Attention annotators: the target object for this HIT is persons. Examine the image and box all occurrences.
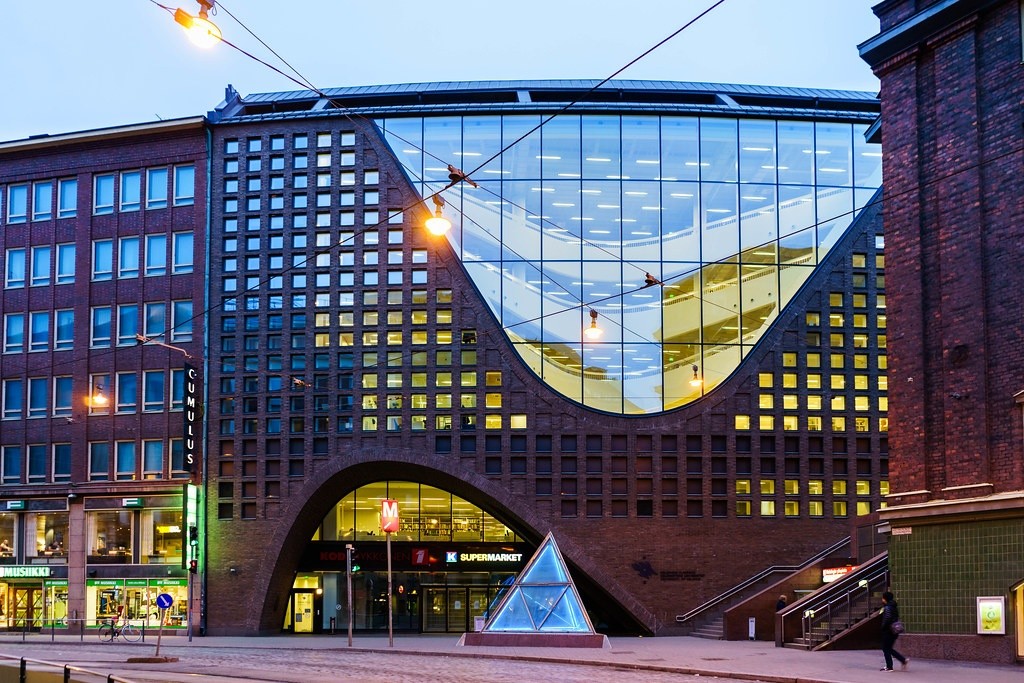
[111,610,121,643]
[0,539,11,553]
[776,594,789,612]
[49,540,61,551]
[876,590,910,672]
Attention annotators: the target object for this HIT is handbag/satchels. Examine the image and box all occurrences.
[889,615,904,634]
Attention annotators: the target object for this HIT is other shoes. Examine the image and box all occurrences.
[880,667,893,671]
[902,658,910,671]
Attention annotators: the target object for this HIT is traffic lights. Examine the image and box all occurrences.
[188,559,198,574]
[189,526,199,547]
[350,549,361,572]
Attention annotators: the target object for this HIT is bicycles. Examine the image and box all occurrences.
[98,616,142,642]
